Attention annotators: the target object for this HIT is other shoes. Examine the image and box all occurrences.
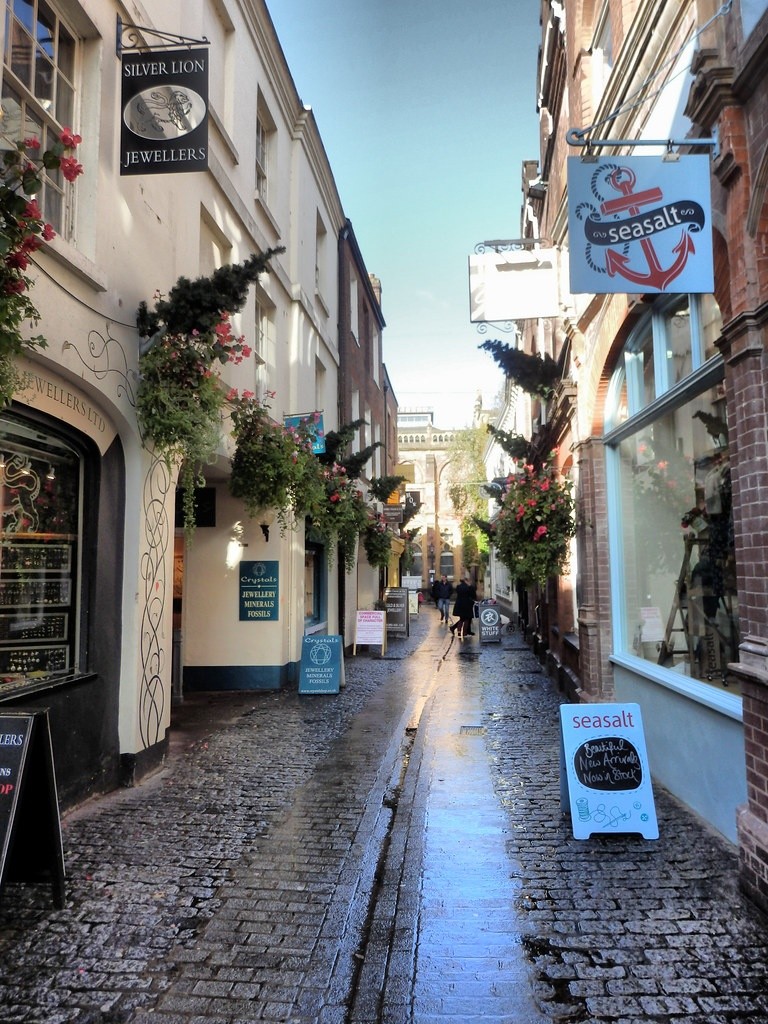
[450,627,454,634]
[458,631,461,636]
[468,632,475,635]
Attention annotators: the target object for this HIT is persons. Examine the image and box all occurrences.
[703,448,738,596]
[416,590,423,607]
[431,574,478,637]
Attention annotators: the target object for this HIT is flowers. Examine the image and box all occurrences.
[135,311,414,575]
[483,446,595,594]
[630,410,730,579]
[0,124,84,411]
